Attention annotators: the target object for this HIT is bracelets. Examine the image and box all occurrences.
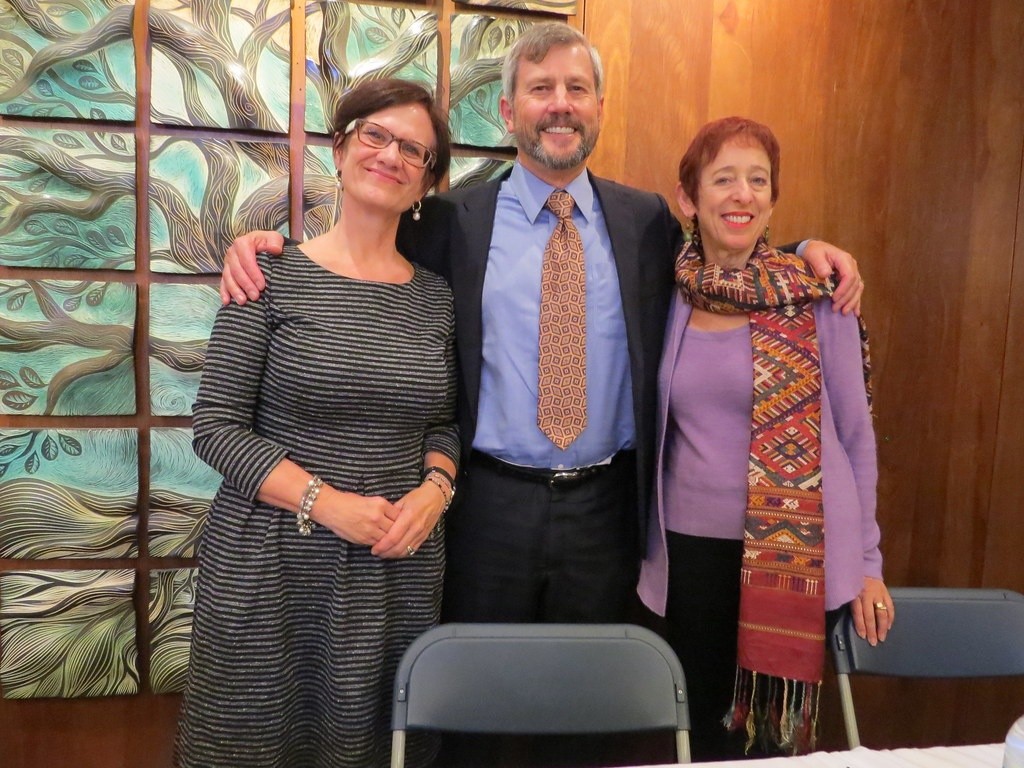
[424,475,454,516]
[423,466,456,491]
[296,474,323,536]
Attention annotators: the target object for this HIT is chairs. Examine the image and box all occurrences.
[390,623,691,768]
[827,587,1024,751]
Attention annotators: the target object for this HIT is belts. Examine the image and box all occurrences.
[469,448,625,494]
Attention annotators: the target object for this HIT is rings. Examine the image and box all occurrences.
[407,546,415,555]
[874,602,886,609]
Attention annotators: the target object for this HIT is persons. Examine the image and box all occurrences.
[221,21,864,768]
[174,78,460,767]
[636,117,894,761]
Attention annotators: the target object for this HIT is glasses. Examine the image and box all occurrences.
[334,119,438,172]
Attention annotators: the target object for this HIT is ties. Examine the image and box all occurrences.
[537,190,587,451]
